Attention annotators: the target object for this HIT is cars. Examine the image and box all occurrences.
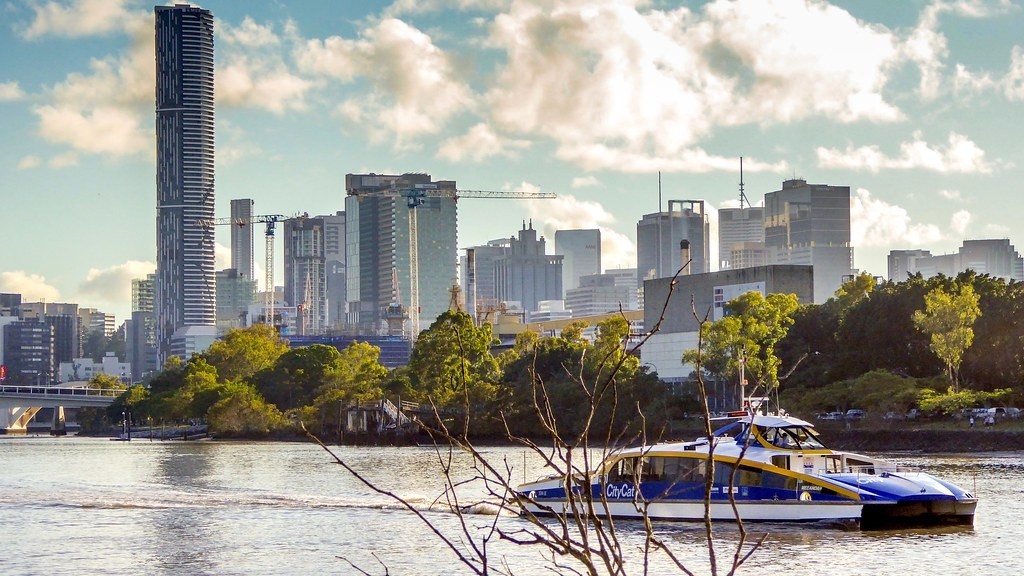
[817,406,1024,421]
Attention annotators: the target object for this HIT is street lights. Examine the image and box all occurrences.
[150,370,153,378]
[129,372,132,387]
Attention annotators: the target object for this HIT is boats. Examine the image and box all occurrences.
[515,343,979,532]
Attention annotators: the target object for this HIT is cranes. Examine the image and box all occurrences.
[346,186,557,358]
[192,211,309,336]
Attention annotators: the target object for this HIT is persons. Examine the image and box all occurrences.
[983,415,994,430]
[969,416,974,430]
[777,432,790,449]
